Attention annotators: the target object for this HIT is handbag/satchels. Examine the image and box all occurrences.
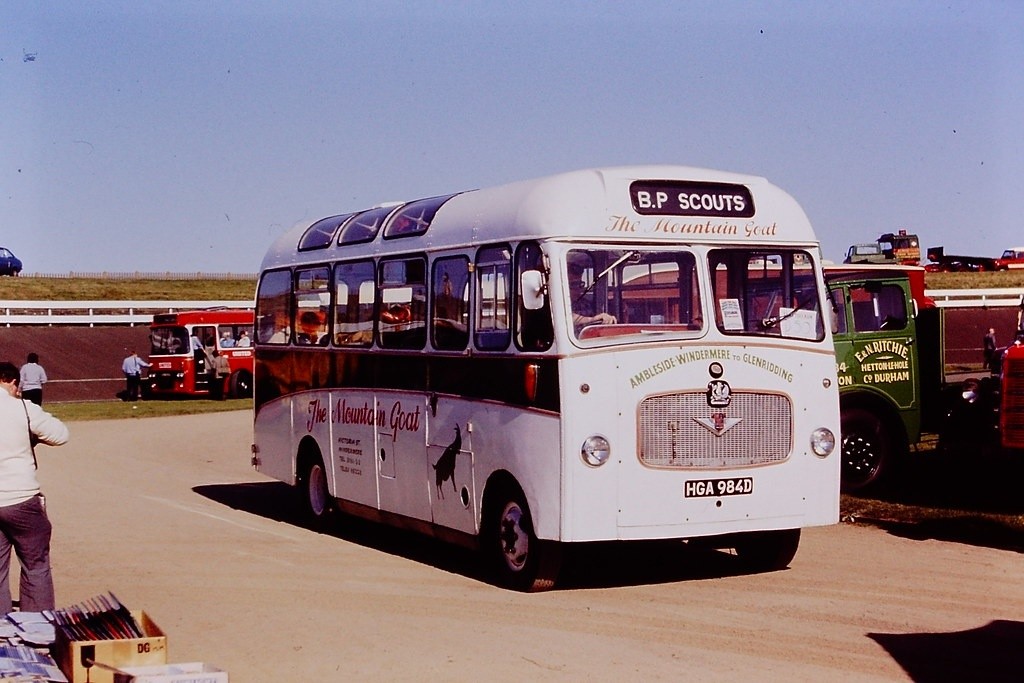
[20,396,39,470]
[137,372,141,385]
[217,366,231,374]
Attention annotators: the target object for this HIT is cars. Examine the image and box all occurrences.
[995,245,1023,270]
[0,247,25,276]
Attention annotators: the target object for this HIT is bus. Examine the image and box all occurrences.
[250,162,845,595]
[147,306,254,401]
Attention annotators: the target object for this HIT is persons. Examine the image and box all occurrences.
[983,328,994,369]
[0,352,69,616]
[122,351,153,401]
[567,262,618,324]
[192,330,250,402]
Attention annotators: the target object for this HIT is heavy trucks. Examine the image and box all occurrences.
[824,268,1024,495]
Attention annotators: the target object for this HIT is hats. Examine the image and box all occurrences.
[239,331,244,334]
[443,272,450,283]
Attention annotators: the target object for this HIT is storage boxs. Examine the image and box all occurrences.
[86,658,228,683]
[52,610,168,683]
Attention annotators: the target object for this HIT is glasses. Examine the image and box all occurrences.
[571,281,585,290]
